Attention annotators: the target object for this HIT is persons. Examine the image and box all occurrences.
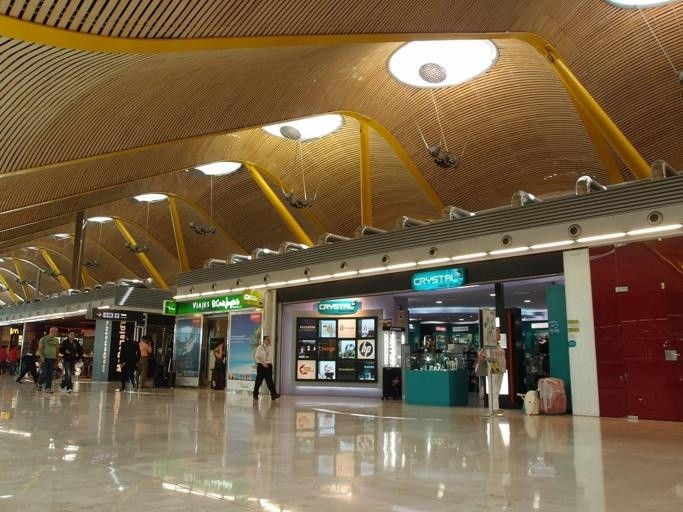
[213,342,228,368]
[15,352,40,385]
[252,336,281,400]
[0,345,9,374]
[478,328,507,410]
[135,335,152,388]
[9,345,21,375]
[33,326,59,394]
[59,329,84,393]
[119,331,142,392]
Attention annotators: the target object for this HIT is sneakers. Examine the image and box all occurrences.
[252,394,257,399]
[13,378,73,394]
[270,393,280,399]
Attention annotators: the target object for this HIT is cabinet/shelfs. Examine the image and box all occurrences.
[404,351,466,407]
[432,331,479,392]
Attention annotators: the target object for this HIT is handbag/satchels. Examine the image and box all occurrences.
[516,389,540,415]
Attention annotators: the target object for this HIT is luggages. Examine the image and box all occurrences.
[538,378,567,414]
